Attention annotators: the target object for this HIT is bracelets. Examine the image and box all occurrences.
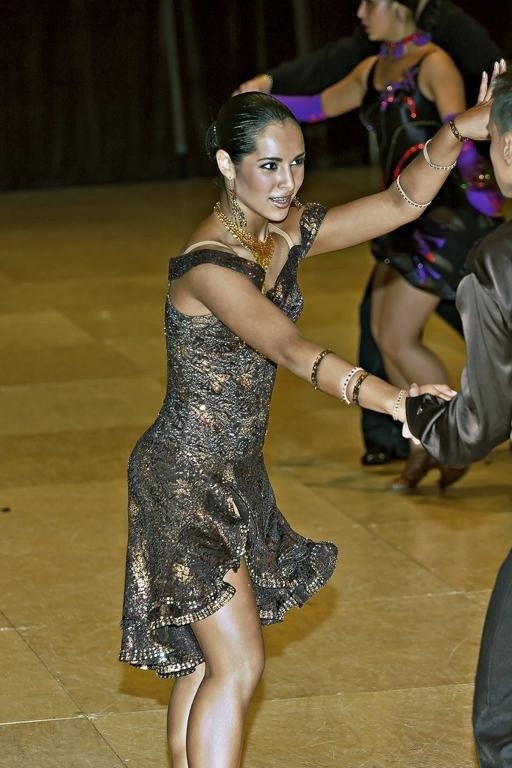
[310,346,407,422]
[447,110,472,146]
[423,133,459,171]
[394,171,433,209]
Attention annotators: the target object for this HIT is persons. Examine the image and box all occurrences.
[241,0,510,467]
[117,53,509,768]
[231,1,506,498]
[470,77,511,767]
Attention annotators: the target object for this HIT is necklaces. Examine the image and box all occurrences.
[212,196,275,295]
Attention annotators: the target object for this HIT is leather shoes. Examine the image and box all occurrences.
[362,449,407,464]
[442,468,467,490]
[392,445,434,489]
